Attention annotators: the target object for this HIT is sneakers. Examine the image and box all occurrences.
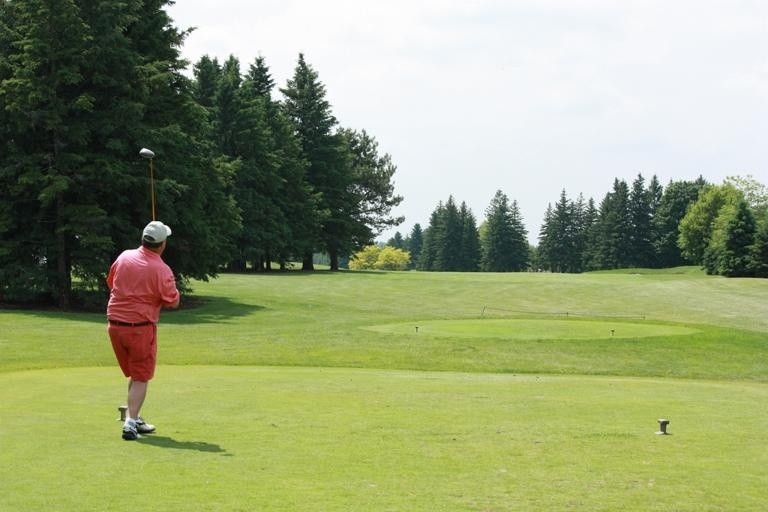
[136,417,155,433]
[122,424,137,440]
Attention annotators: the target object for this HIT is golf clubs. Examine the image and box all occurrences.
[140,148,156,221]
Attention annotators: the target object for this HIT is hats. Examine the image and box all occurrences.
[143,220,172,244]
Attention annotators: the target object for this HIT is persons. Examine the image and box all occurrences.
[107,221,180,440]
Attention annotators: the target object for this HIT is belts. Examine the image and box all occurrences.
[110,321,147,326]
